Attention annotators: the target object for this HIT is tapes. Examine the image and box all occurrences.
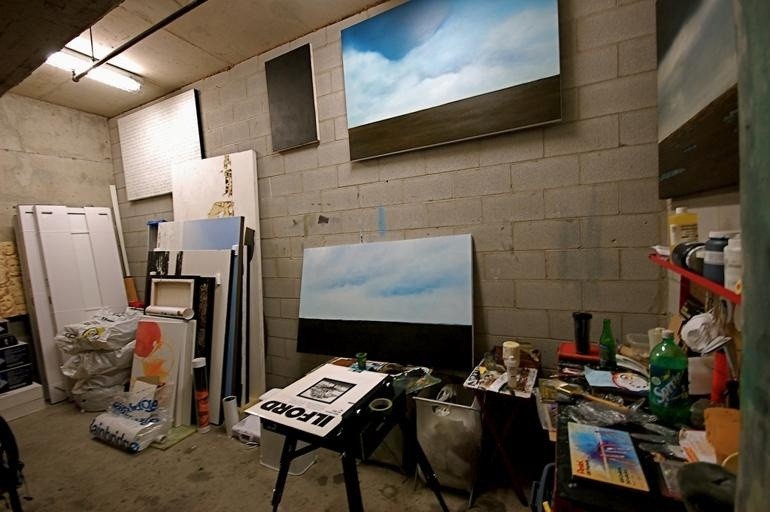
[368,397,393,417]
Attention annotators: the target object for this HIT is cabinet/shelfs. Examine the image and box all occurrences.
[528,343,740,512]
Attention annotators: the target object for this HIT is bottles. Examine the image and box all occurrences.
[711,348,730,403]
[670,241,706,273]
[669,206,699,265]
[648,328,690,426]
[702,231,729,283]
[503,340,520,388]
[599,318,617,371]
[723,233,743,292]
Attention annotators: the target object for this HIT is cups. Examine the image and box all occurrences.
[572,311,593,355]
[354,352,367,371]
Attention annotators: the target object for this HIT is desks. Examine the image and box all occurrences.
[462,345,541,420]
[238,355,453,511]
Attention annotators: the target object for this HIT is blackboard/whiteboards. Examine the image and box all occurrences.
[264,44,319,153]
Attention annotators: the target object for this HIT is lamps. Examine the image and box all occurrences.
[41,51,142,94]
